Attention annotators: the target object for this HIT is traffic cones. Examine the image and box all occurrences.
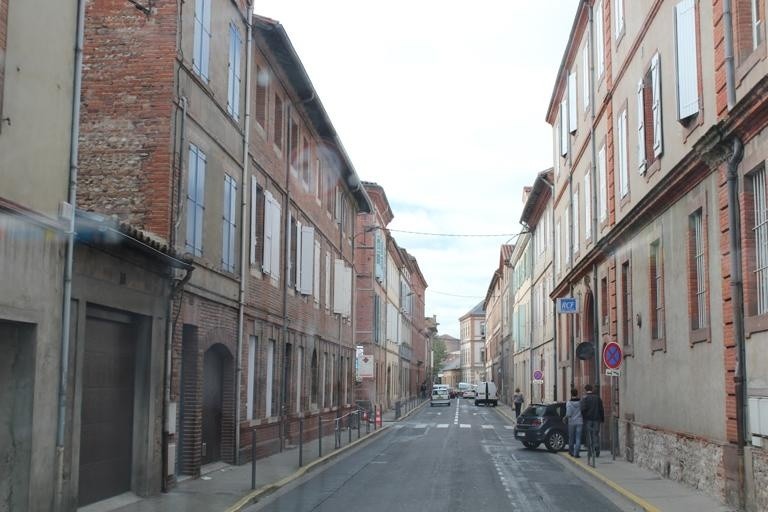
[363,409,367,421]
[375,407,381,425]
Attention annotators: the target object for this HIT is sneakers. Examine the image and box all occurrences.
[596,447,600,457]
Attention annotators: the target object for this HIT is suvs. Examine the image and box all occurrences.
[510,399,590,453]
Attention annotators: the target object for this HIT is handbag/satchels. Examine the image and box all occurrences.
[562,417,568,425]
[512,403,515,410]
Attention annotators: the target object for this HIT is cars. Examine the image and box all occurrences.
[429,381,499,408]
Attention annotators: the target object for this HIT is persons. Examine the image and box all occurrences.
[511,387,525,419]
[420,382,427,399]
[581,383,605,458]
[562,387,585,458]
